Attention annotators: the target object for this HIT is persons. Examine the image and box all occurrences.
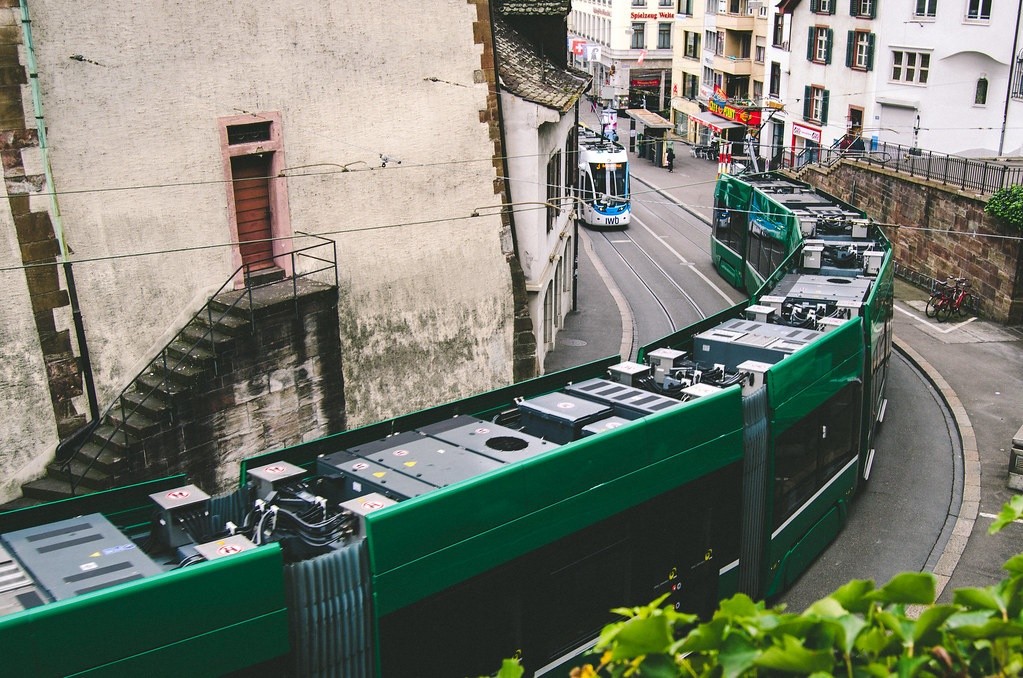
[666,147,675,173]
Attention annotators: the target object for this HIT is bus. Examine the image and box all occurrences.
[0,168,892,678]
[576,123,631,229]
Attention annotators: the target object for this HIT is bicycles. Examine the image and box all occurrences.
[925,276,973,323]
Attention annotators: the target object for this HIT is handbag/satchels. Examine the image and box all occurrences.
[671,154,675,158]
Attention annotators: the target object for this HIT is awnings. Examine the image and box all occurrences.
[689,112,746,134]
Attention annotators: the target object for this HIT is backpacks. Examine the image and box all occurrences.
[613,135,618,141]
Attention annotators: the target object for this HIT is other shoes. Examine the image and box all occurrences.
[669,170,673,172]
